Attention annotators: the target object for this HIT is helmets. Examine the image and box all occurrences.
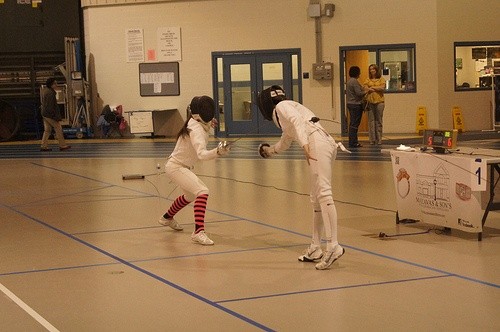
[257,85,286,121]
[190,96,216,124]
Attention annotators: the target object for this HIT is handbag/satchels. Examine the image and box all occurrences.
[362,100,370,111]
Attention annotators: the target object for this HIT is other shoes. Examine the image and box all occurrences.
[370,142,374,145]
[40,148,53,151]
[378,143,381,144]
[60,146,71,150]
[349,144,360,147]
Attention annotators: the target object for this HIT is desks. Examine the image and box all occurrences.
[380,148,500,242]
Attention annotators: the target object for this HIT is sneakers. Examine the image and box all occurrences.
[315,245,345,270]
[191,230,214,245]
[298,246,323,263]
[158,215,183,231]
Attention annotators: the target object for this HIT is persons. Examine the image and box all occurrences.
[41,77,71,150]
[362,64,386,145]
[158,95,231,246]
[257,85,344,270]
[345,66,369,147]
